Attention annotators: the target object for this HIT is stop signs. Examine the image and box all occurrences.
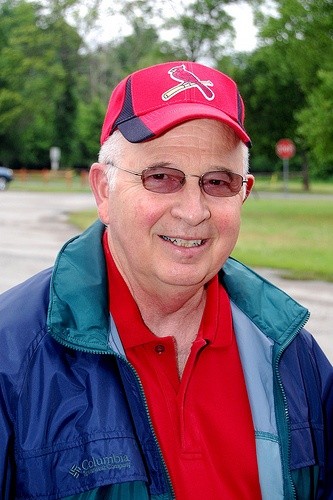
[274,138,296,160]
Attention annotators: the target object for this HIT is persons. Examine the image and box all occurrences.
[1,61,333,499]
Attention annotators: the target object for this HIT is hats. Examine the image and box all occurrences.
[102,62,250,147]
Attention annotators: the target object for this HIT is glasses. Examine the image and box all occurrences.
[103,162,248,198]
